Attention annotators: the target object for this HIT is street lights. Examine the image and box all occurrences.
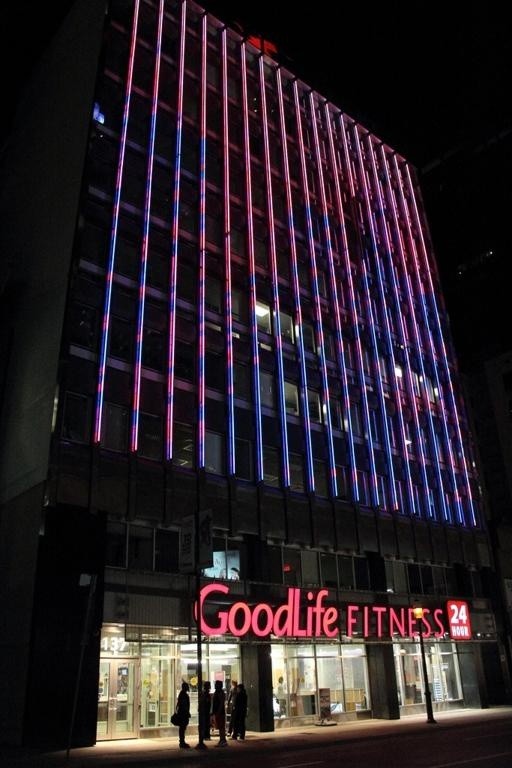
[410,596,438,726]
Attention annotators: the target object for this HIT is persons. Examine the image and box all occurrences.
[198,681,212,751]
[225,680,242,736]
[209,680,228,747]
[278,676,287,716]
[176,683,190,748]
[229,684,248,741]
